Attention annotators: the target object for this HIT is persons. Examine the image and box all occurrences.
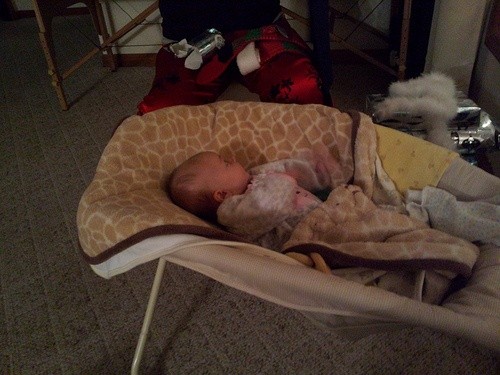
[167,151,480,306]
[137,0,324,117]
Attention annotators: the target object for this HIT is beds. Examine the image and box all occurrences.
[73,100,499,350]
